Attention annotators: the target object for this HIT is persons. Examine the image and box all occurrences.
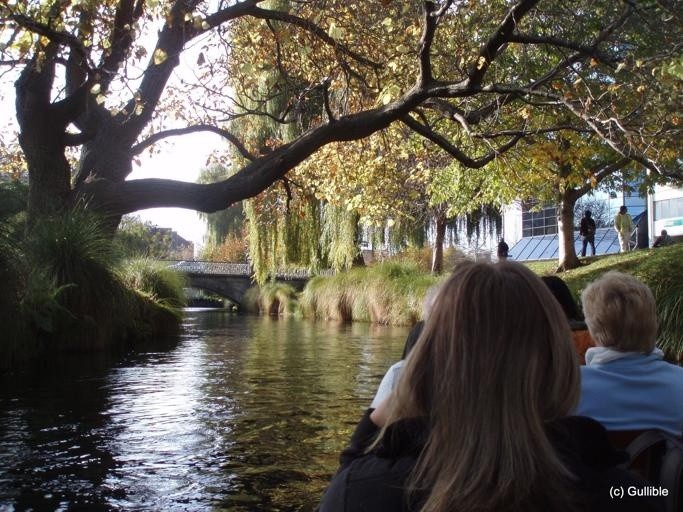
[579,210,596,257]
[613,207,634,253]
[651,230,677,248]
[497,237,509,261]
